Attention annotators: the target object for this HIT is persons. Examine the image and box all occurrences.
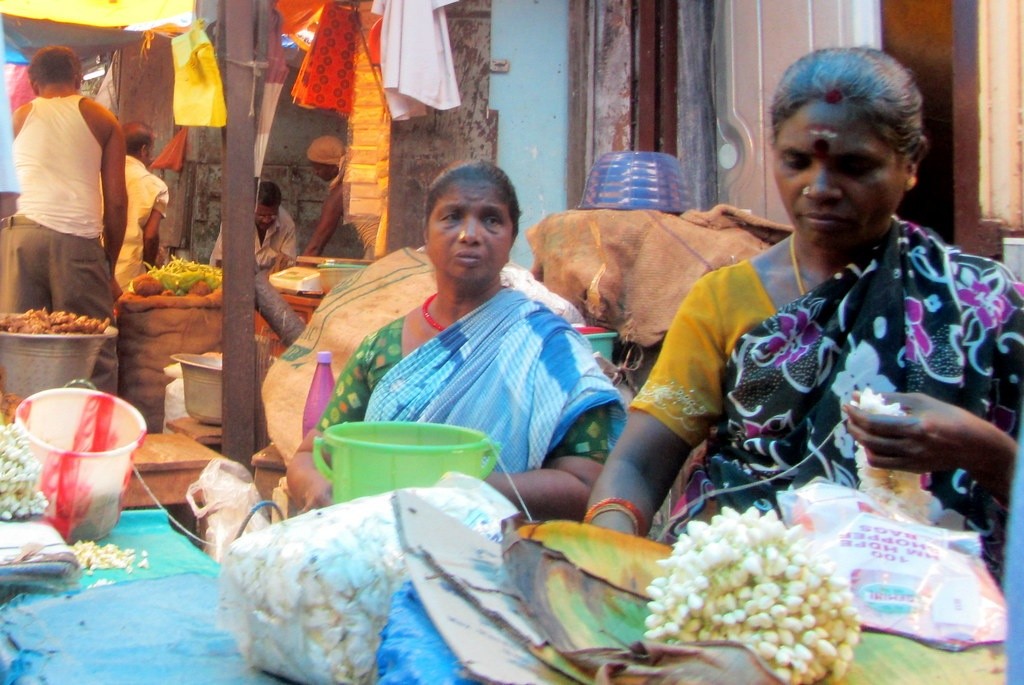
[1,45,130,404]
[299,135,381,267]
[207,180,297,276]
[99,122,169,298]
[585,43,1024,539]
[286,158,610,522]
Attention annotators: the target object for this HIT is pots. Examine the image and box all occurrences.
[170,353,277,424]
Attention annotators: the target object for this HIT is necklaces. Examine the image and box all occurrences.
[422,292,445,333]
[790,230,807,295]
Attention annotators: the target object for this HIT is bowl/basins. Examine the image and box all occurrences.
[577,151,693,213]
[317,264,368,295]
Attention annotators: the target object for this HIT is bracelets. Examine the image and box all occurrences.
[583,497,647,540]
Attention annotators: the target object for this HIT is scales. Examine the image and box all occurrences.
[268,255,376,296]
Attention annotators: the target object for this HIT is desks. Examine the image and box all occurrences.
[121,431,225,506]
[166,414,224,443]
[0,508,284,685]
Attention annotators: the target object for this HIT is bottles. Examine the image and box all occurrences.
[302,352,337,439]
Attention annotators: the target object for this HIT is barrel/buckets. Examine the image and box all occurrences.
[314,422,500,504]
[0,313,119,402]
[574,326,618,362]
[16,388,148,543]
